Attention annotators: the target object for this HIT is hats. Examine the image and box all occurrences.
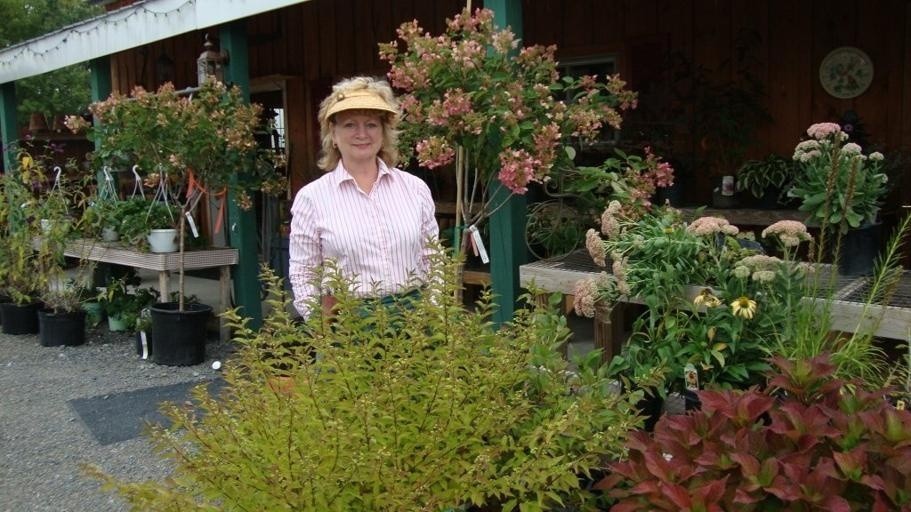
[324,87,396,123]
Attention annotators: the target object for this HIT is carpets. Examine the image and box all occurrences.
[65,376,243,447]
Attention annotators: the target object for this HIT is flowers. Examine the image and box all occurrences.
[365,0,674,320]
[63,81,290,311]
[569,197,816,406]
[792,109,910,236]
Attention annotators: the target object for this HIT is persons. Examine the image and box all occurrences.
[288,77,445,373]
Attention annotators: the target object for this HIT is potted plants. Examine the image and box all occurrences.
[2,140,91,348]
[19,64,95,138]
[733,154,789,210]
[674,49,776,208]
[41,167,177,254]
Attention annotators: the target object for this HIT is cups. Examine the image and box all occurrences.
[722,175,734,196]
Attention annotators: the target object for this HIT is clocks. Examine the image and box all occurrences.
[818,46,874,100]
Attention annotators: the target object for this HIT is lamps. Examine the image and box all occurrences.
[153,52,177,83]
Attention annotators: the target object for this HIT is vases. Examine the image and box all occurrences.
[150,302,212,366]
